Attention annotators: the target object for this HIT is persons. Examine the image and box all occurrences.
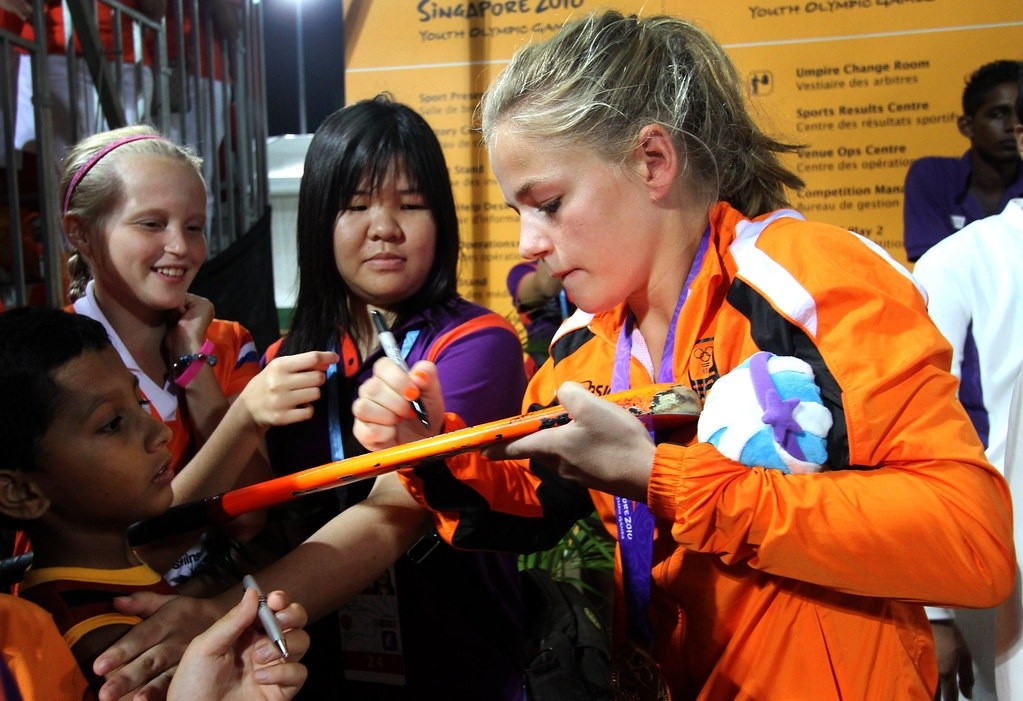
[1,300,182,671]
[0,591,316,700]
[55,122,279,562]
[92,89,539,701]
[913,92,1023,699]
[482,5,1016,700]
[505,251,581,383]
[902,56,1023,263]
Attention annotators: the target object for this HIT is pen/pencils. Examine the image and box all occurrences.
[241,574,290,659]
[370,308,432,433]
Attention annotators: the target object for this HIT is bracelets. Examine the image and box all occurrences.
[168,351,217,381]
[173,338,216,388]
